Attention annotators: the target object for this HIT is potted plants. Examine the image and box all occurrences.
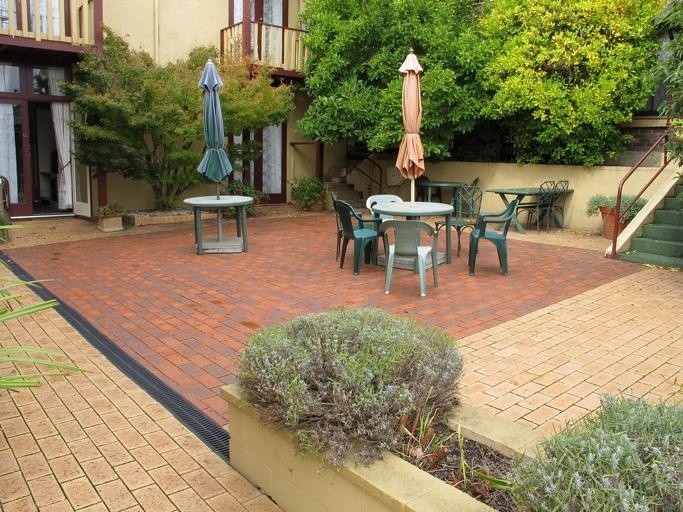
[585,191,647,240]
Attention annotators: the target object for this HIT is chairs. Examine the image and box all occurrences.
[376,219,438,298]
[331,199,381,277]
[372,201,455,272]
[365,193,404,261]
[409,173,582,277]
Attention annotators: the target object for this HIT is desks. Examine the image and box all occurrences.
[181,194,255,255]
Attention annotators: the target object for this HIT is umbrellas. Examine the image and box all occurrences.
[371,45,446,273]
[193,55,245,254]
